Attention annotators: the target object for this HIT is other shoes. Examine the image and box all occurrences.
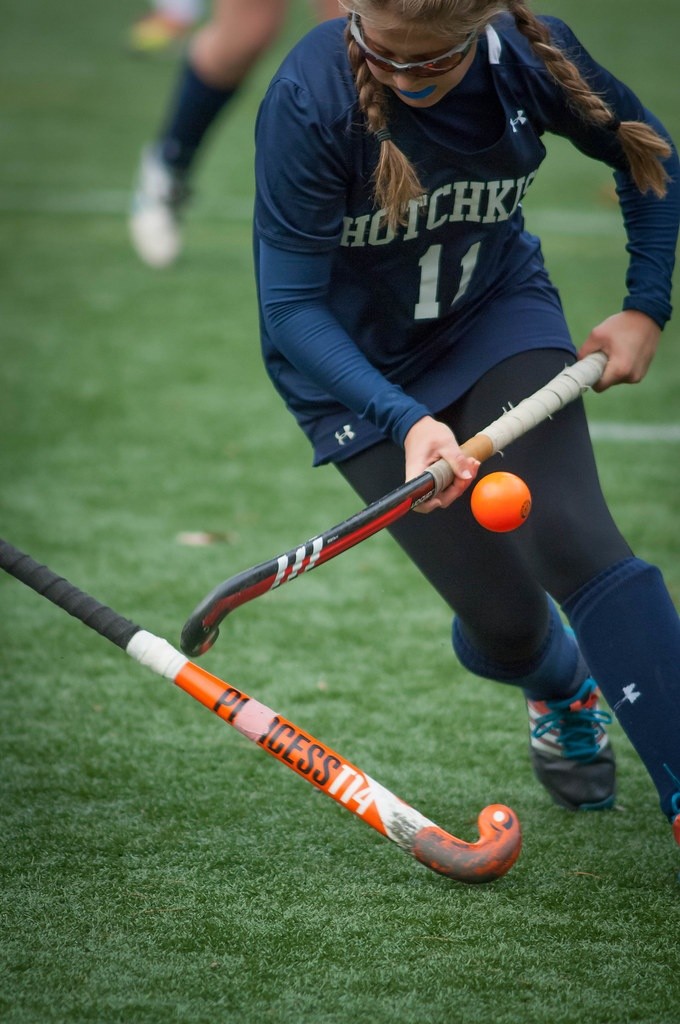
[132,174,184,262]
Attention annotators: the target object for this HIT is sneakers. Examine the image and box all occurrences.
[672,816,680,845]
[526,629,616,811]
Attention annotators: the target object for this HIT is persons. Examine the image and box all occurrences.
[249,0,680,845]
[129,0,353,270]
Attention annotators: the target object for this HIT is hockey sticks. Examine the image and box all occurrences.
[0,538,525,886]
[178,349,609,660]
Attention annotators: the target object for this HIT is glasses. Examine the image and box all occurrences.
[350,9,477,77]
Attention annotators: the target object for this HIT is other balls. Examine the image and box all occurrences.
[468,470,535,534]
